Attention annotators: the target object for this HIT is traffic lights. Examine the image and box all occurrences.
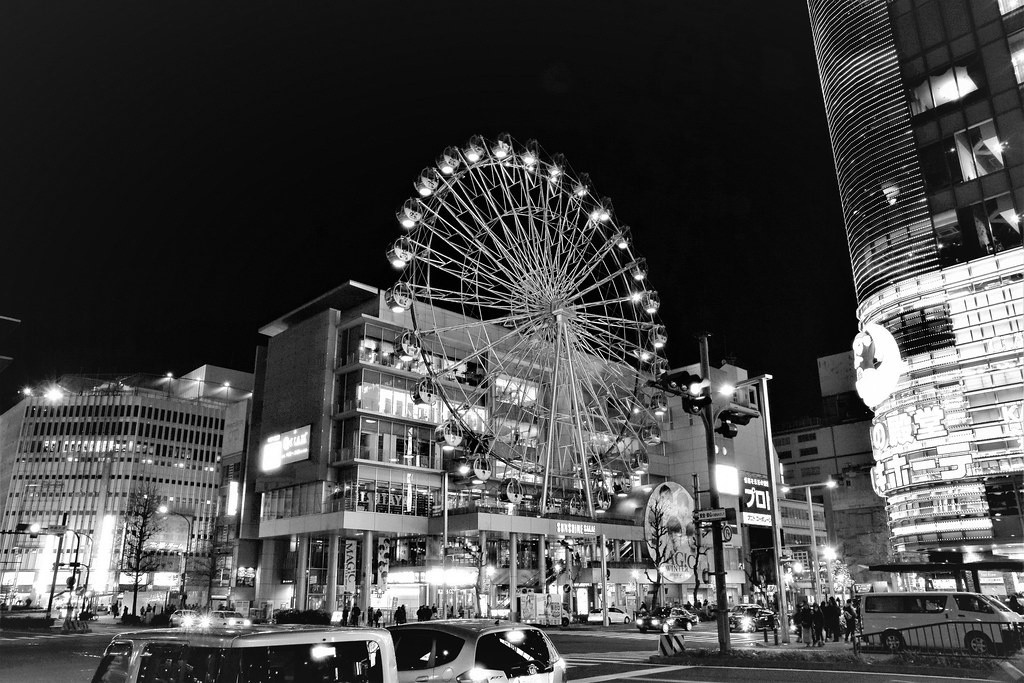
[661,370,704,396]
[715,423,738,438]
[720,411,760,426]
[682,396,712,414]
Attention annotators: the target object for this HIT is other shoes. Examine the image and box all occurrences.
[832,639,839,642]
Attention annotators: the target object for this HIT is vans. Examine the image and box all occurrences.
[92,623,399,683]
[855,592,1024,654]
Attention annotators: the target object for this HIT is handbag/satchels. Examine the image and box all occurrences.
[843,611,852,619]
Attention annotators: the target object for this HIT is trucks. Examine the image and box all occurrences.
[516,594,574,627]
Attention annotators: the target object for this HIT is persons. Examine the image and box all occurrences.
[141,604,177,622]
[795,597,857,647]
[773,602,779,612]
[108,603,128,618]
[184,603,235,610]
[1009,596,1023,613]
[417,604,464,622]
[362,606,385,628]
[358,492,402,505]
[572,552,581,566]
[394,605,406,625]
[687,599,708,610]
[548,558,553,575]
[639,602,660,610]
[343,603,361,627]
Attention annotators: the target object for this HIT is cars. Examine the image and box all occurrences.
[728,607,773,633]
[385,619,567,683]
[208,611,250,629]
[636,606,699,633]
[728,604,762,617]
[588,607,631,625]
[168,610,202,627]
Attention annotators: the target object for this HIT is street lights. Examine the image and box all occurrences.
[159,506,190,608]
[781,481,836,606]
